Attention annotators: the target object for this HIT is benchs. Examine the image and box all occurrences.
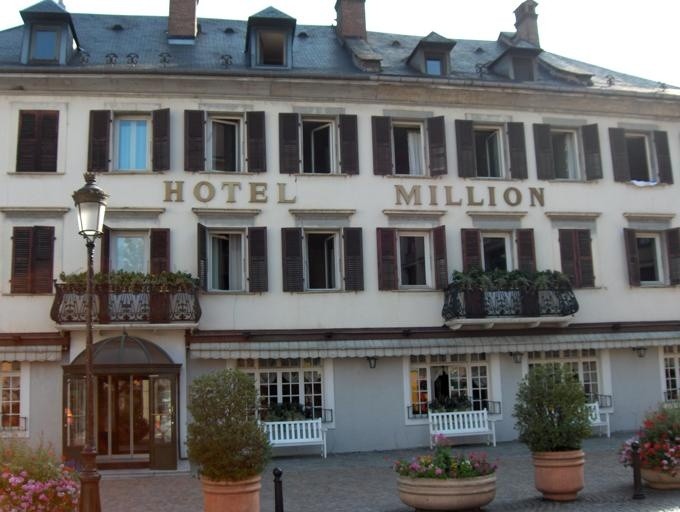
[428,408,496,451]
[569,401,610,438]
[256,417,328,459]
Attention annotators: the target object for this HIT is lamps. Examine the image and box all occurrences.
[631,347,647,357]
[509,351,523,363]
[367,358,378,368]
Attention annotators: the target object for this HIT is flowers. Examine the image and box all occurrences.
[617,398,680,477]
[0,431,81,512]
[383,433,500,481]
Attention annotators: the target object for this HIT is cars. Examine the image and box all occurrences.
[449,378,487,407]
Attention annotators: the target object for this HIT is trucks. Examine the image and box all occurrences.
[412,372,427,412]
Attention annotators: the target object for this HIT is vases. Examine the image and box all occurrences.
[397,472,497,512]
[639,467,680,490]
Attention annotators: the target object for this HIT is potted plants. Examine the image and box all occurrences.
[428,391,471,413]
[262,402,304,422]
[511,362,593,501]
[60,268,201,293]
[182,368,276,512]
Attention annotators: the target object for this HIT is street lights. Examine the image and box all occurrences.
[70,172,111,511]
